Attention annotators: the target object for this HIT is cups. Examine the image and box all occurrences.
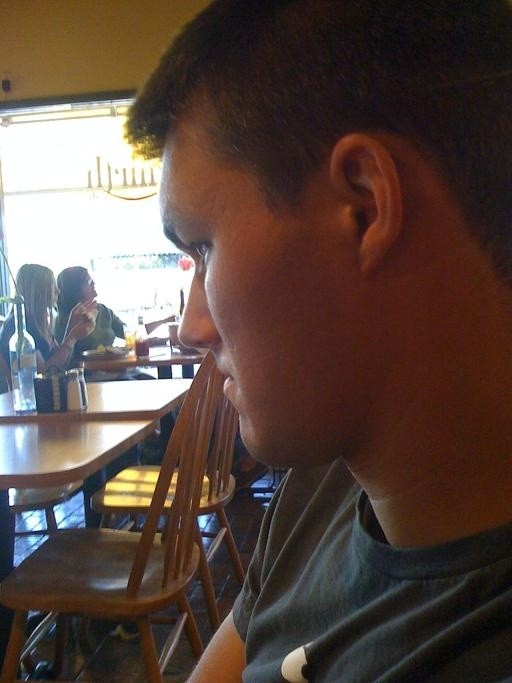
[34,377,53,412]
[169,323,179,346]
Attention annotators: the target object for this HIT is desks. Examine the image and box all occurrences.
[1,379,198,479]
[2,420,161,492]
[82,345,206,377]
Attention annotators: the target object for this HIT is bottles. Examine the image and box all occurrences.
[65,366,89,411]
[135,315,149,356]
[179,288,186,316]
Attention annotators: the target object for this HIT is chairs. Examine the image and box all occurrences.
[4,347,248,682]
[3,481,85,570]
[1,358,18,393]
[88,379,249,632]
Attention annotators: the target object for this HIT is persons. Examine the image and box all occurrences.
[125,0,512,683]
[55,265,177,381]
[1,263,100,391]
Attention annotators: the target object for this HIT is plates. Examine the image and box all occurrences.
[81,346,129,358]
